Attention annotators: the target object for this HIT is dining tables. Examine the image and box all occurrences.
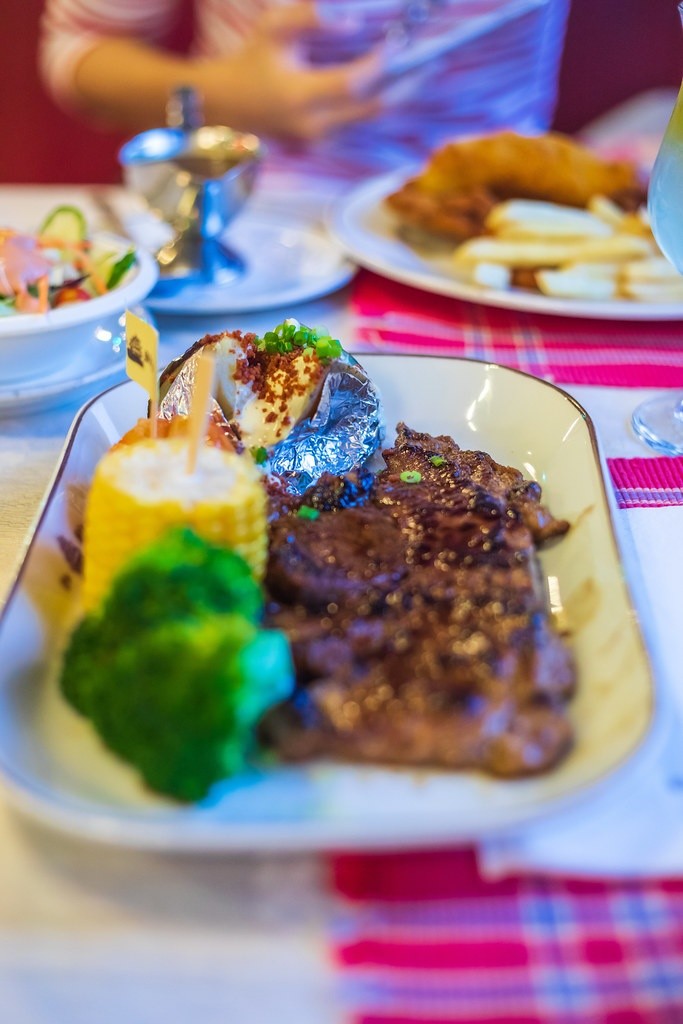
[4,180,682,1024]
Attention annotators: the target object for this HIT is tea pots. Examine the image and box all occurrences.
[116,83,270,284]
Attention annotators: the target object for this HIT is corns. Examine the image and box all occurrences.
[82,443,266,616]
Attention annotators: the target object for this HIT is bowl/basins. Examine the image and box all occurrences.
[1,225,155,380]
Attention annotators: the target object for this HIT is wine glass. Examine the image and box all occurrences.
[634,77,683,456]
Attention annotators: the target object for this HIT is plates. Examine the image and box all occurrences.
[2,355,659,857]
[1,309,155,421]
[324,155,683,321]
[83,199,357,314]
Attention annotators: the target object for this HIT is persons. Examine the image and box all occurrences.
[36,2,582,188]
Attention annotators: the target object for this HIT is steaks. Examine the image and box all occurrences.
[266,423,577,774]
